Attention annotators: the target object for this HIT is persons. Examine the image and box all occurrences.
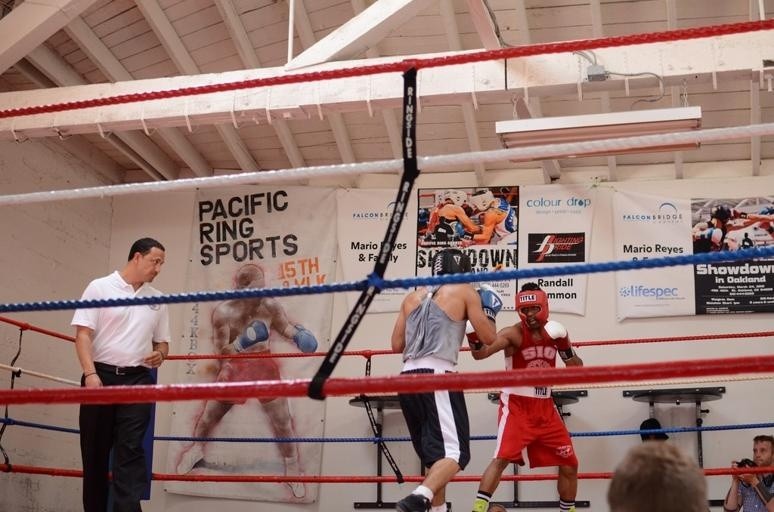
[391,249,501,512]
[602,440,710,512]
[68,237,171,511]
[639,417,669,444]
[693,202,774,255]
[722,434,774,511]
[463,282,587,512]
[174,264,318,498]
[487,504,508,512]
[418,186,519,247]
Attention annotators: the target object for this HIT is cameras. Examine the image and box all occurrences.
[737,459,758,468]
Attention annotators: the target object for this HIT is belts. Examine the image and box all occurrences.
[93,362,145,376]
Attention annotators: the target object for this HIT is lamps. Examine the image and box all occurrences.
[495,79,702,162]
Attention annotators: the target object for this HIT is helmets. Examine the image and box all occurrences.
[516,290,549,327]
[431,251,472,276]
[759,206,774,215]
[711,207,731,222]
[470,191,495,210]
[444,189,467,206]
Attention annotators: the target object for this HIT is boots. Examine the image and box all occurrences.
[284,455,306,497]
[177,447,204,475]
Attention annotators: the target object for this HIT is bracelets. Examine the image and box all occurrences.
[155,348,165,363]
[82,372,99,378]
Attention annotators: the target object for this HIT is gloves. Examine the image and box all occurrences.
[696,228,722,243]
[284,323,317,353]
[757,221,770,229]
[477,284,503,320]
[234,320,270,353]
[540,321,576,361]
[465,320,483,350]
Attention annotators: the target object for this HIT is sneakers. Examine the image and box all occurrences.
[396,495,431,512]
[472,498,489,512]
